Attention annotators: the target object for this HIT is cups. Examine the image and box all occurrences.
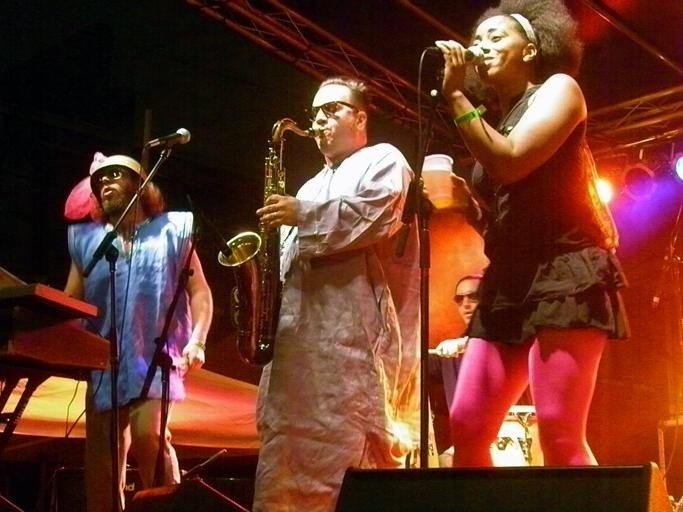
[421,152,456,211]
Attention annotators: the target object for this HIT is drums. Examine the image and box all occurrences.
[488,403,546,467]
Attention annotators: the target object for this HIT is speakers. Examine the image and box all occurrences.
[335,462,674,512]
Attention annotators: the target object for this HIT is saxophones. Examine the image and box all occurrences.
[217,119,322,369]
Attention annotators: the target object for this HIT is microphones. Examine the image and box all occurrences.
[145,127,192,148]
[189,200,233,258]
[424,42,485,67]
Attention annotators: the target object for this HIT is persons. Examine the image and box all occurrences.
[436,274,485,359]
[252,75,416,512]
[64,154,214,512]
[418,0,631,468]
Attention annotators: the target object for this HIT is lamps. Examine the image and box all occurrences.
[589,138,683,211]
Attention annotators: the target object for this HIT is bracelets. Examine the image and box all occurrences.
[454,105,487,127]
[190,338,207,351]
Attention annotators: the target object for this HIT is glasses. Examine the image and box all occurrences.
[454,293,480,305]
[304,101,360,122]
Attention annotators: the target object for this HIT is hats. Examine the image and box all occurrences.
[90,154,147,199]
